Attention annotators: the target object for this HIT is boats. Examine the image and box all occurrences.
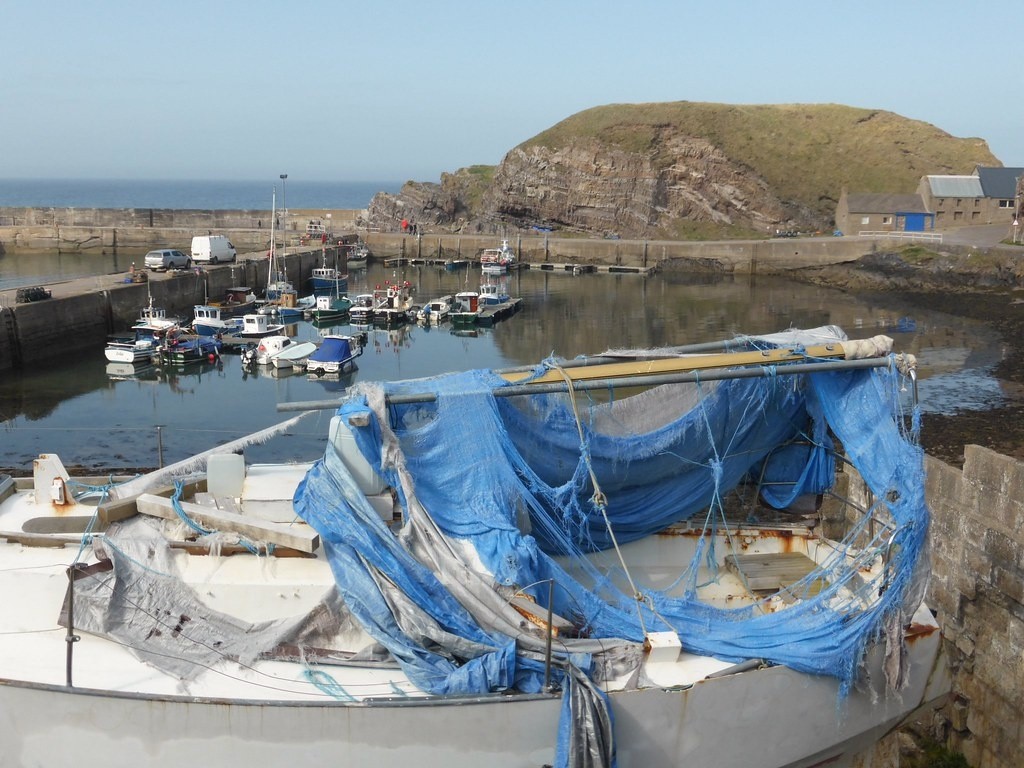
[347,239,369,270]
[104,183,518,378]
[309,245,350,290]
[1,318,959,768]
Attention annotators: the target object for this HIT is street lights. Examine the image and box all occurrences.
[153,423,168,469]
[280,171,288,255]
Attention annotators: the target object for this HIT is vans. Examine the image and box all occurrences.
[190,234,237,265]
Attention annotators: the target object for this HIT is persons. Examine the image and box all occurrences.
[413,223,418,235]
[409,223,413,235]
[257,220,262,229]
[276,218,280,230]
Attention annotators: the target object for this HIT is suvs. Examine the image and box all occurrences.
[144,248,192,273]
